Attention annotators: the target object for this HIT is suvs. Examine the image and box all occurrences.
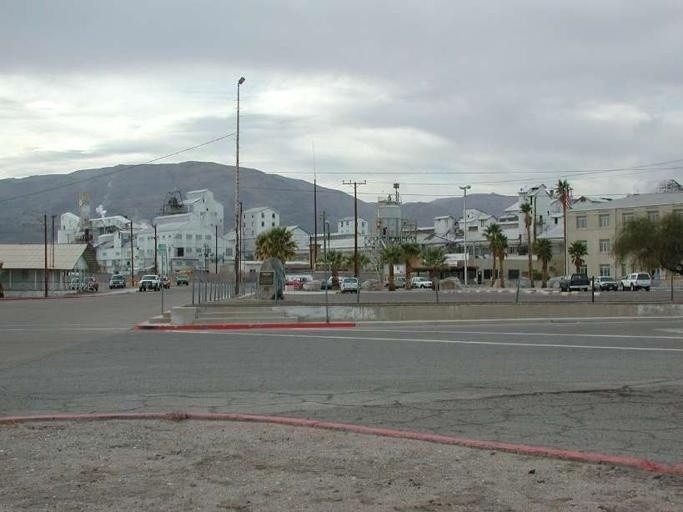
[558,272,588,292]
[617,272,651,293]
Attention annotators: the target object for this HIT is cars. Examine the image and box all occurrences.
[68,270,189,294]
[286,274,433,294]
[590,275,618,292]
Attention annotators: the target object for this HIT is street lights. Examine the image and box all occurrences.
[151,223,157,275]
[459,183,471,287]
[235,75,246,298]
[125,219,135,287]
[210,223,218,275]
